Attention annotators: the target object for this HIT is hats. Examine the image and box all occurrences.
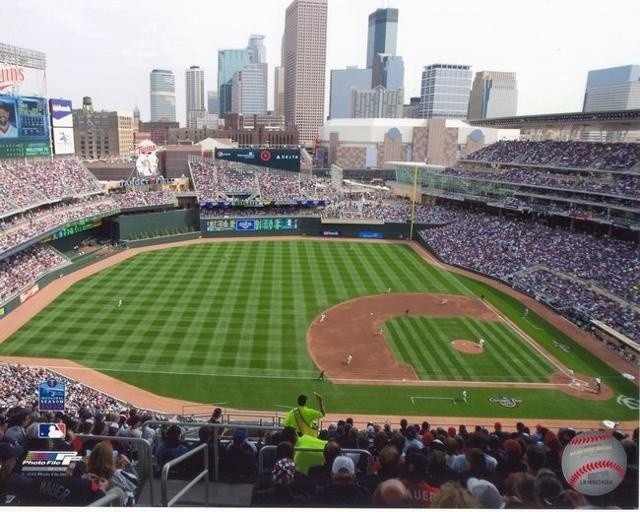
[0,442,25,463]
[331,455,355,479]
[467,477,500,507]
[271,457,296,486]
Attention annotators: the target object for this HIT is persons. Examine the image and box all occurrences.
[320,311,327,324]
[405,309,409,318]
[116,297,123,309]
[369,310,375,320]
[0,358,639,509]
[346,353,354,365]
[0,154,415,304]
[414,139,640,358]
[379,326,385,338]
[1,98,19,139]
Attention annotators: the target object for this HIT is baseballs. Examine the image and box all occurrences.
[561,432,627,496]
